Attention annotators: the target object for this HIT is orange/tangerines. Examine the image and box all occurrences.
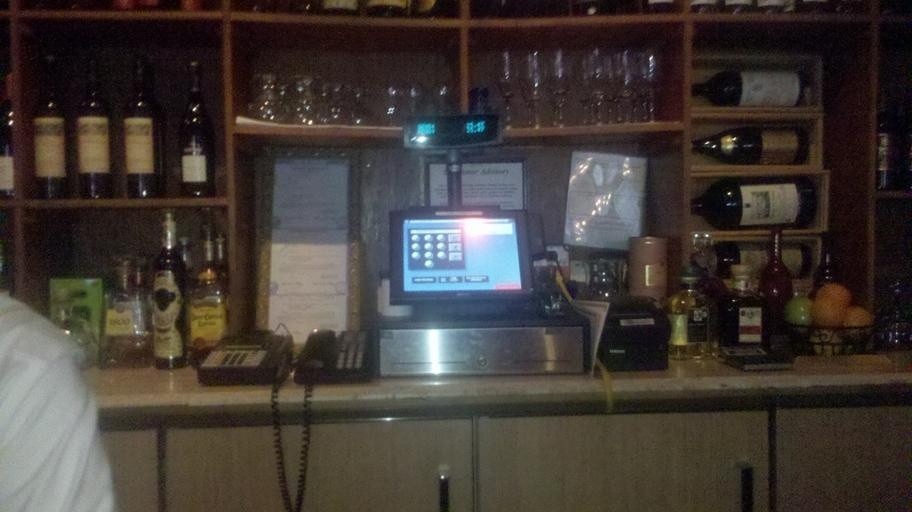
[812,296,849,327]
[845,304,877,327]
[784,295,814,326]
[815,283,853,304]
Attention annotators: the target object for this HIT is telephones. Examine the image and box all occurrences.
[293,329,379,383]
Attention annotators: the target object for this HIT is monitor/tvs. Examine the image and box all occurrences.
[388,211,530,305]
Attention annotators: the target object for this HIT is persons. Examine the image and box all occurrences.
[1,291,115,512]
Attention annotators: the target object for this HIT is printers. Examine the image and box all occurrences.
[597,296,671,371]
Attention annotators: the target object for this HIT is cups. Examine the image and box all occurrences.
[403,84,424,126]
[291,74,324,124]
[376,80,407,126]
[426,82,450,118]
[243,71,291,125]
[326,80,361,128]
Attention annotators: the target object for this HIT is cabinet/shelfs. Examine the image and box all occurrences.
[159,413,474,512]
[98,424,161,511]
[473,410,772,512]
[772,407,912,512]
[0,1,912,352]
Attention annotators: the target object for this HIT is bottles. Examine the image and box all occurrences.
[877,87,897,191]
[690,126,807,164]
[759,229,794,314]
[691,66,806,107]
[131,267,151,367]
[152,214,185,367]
[178,60,217,198]
[815,253,845,286]
[665,276,709,360]
[691,176,818,228]
[0,114,14,198]
[99,267,141,369]
[31,89,67,198]
[72,79,116,201]
[187,223,228,366]
[122,70,169,199]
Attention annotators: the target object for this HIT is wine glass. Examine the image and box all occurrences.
[525,48,547,128]
[583,47,610,128]
[551,47,576,132]
[495,48,520,133]
[640,45,667,124]
[613,48,639,125]
